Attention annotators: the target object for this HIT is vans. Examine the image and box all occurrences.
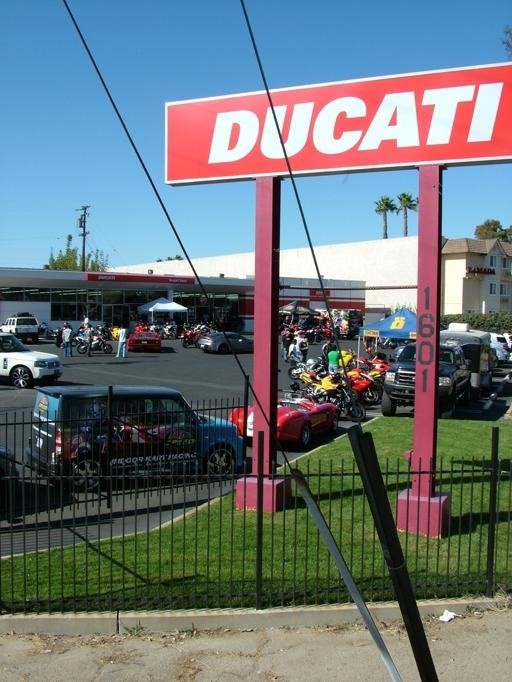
[25,385,243,479]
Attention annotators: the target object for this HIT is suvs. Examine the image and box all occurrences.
[0,314,40,340]
[381,339,472,418]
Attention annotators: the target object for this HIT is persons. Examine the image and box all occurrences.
[62,314,176,358]
[279,323,340,375]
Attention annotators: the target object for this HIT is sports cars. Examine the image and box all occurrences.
[229,395,341,446]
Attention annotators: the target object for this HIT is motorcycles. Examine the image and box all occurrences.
[142,320,211,347]
[76,323,123,356]
[282,320,394,420]
[54,323,69,346]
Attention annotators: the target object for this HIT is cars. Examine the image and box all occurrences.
[1,444,20,493]
[200,328,252,354]
[490,331,511,366]
[128,329,162,352]
[0,330,63,388]
